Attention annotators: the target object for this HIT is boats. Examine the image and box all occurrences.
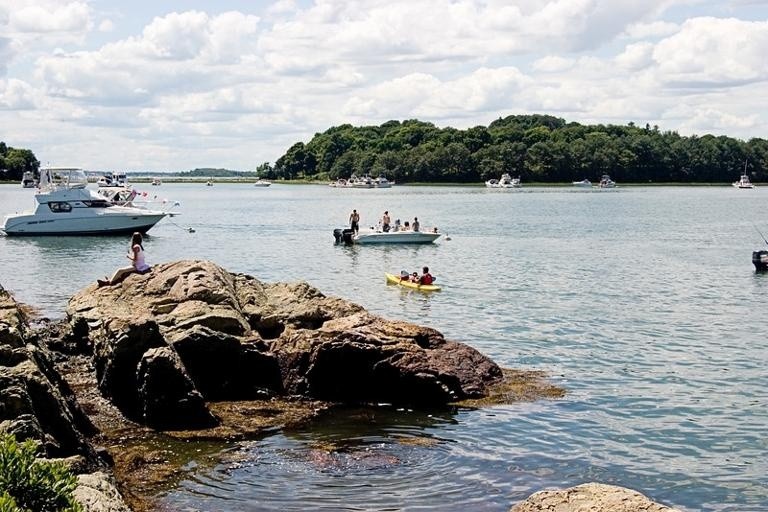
[333,228,440,243]
[750,221,767,273]
[99,169,128,186]
[573,179,592,188]
[499,174,513,189]
[385,271,443,292]
[0,163,166,238]
[254,182,271,187]
[207,183,213,186]
[485,179,501,189]
[598,175,616,188]
[732,175,756,189]
[151,181,156,185]
[329,173,395,188]
[21,172,34,188]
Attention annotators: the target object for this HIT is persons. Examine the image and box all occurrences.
[410,272,417,282]
[412,217,420,232]
[349,210,359,236]
[402,221,410,231]
[97,231,151,287]
[414,266,432,284]
[382,211,390,232]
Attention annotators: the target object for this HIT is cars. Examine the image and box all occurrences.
[510,179,524,187]
